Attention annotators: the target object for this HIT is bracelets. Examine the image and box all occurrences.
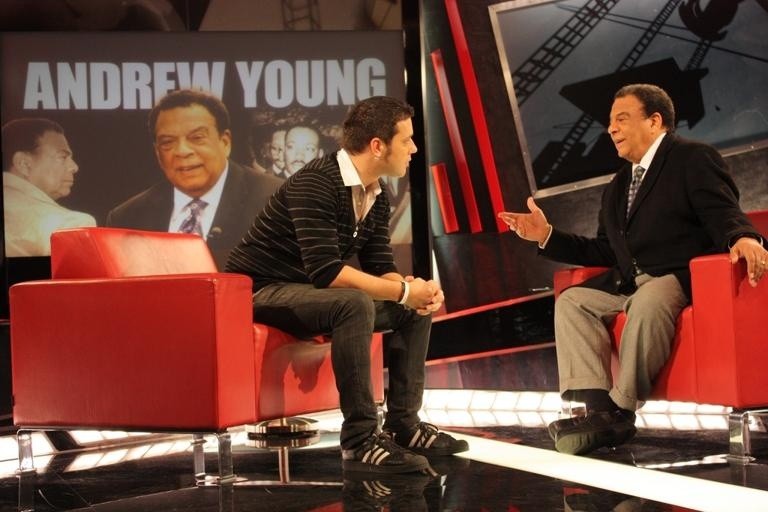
[397,280,409,305]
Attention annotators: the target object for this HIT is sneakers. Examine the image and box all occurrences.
[341,431,429,472]
[392,423,469,456]
[342,473,429,499]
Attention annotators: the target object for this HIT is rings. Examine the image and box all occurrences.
[761,260,766,265]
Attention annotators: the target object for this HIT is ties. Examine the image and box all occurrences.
[177,199,207,236]
[626,166,645,218]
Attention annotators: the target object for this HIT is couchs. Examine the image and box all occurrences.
[547,211,767,465]
[7,226,384,488]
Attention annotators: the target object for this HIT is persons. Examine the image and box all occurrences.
[221,96,470,475]
[105,87,287,272]
[3,111,98,259]
[496,84,768,454]
[241,107,412,246]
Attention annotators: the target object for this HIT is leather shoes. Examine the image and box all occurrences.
[548,409,636,455]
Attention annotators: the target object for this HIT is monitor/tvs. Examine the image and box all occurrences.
[487,0,768,198]
